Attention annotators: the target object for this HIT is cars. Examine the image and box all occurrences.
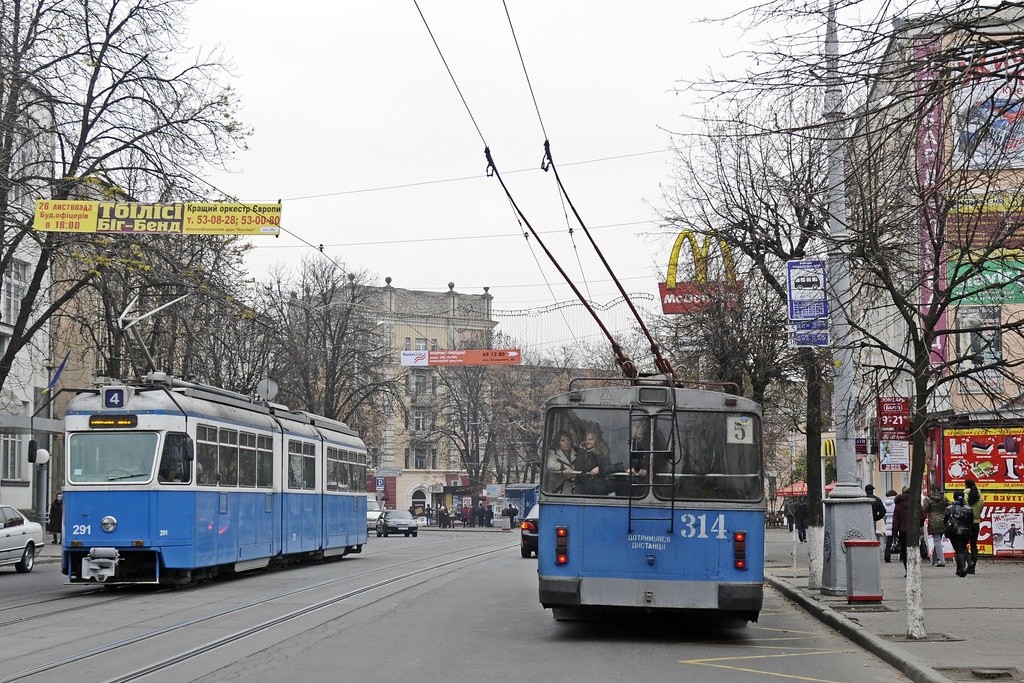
[520,502,539,559]
[366,498,383,533]
[0,504,45,573]
[375,509,419,537]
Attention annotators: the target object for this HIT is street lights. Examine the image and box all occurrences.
[35,448,50,543]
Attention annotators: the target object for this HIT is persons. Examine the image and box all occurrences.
[409,507,417,519]
[424,504,435,525]
[197,462,209,485]
[793,497,808,542]
[475,502,494,527]
[865,483,930,577]
[964,479,985,573]
[784,500,795,532]
[439,504,456,529]
[944,489,974,577]
[922,482,951,567]
[573,423,610,494]
[502,502,519,529]
[46,492,63,545]
[547,429,585,496]
[105,450,142,477]
[461,501,475,527]
[611,414,667,494]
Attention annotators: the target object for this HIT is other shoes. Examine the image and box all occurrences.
[966,562,974,573]
[937,564,945,566]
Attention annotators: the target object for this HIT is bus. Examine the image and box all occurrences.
[26,280,370,592]
[482,137,768,633]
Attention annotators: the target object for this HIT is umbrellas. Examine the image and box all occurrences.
[775,480,807,501]
[825,482,836,493]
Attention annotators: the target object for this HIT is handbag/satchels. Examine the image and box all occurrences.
[944,505,958,538]
[890,536,902,554]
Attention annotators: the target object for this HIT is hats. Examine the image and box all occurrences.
[865,484,875,490]
[886,489,897,496]
[931,483,939,491]
